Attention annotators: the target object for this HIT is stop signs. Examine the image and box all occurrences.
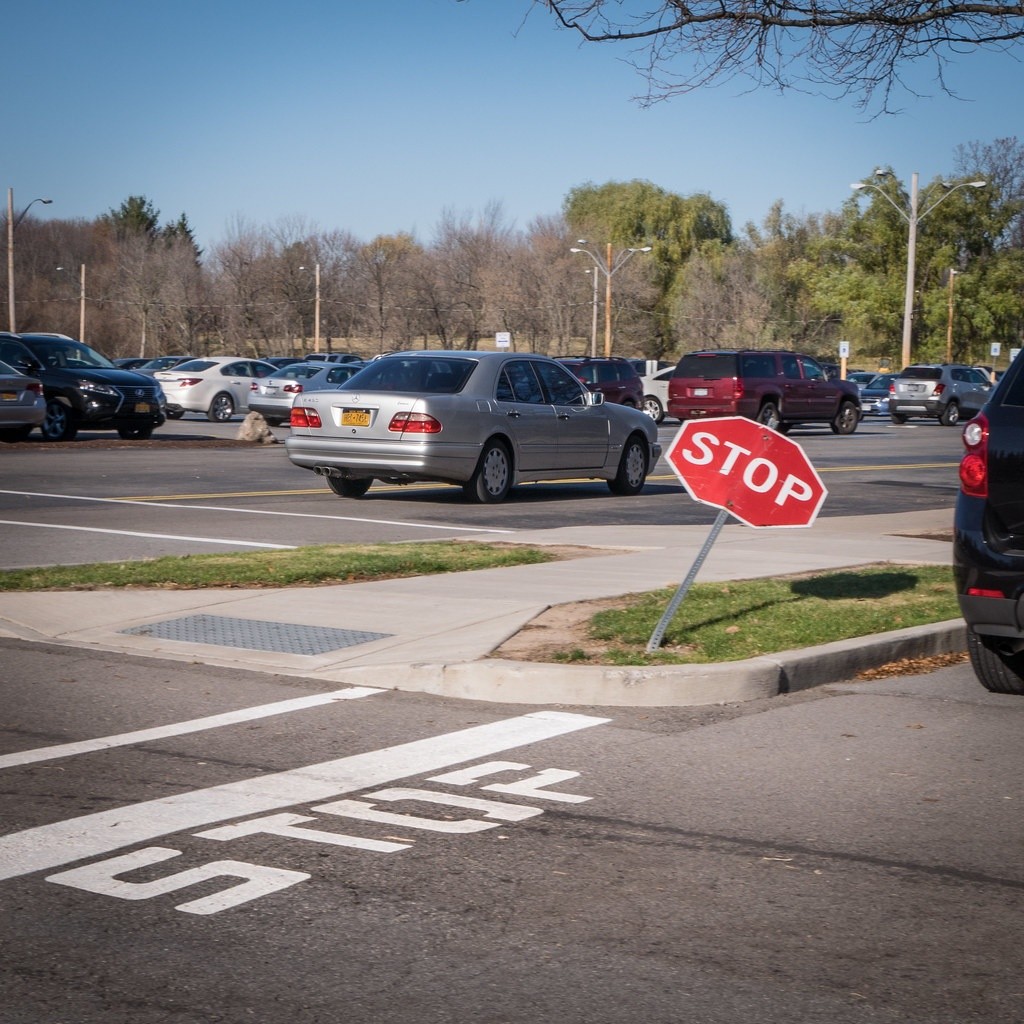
[663,413,828,530]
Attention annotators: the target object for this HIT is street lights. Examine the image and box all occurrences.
[584,264,599,360]
[6,185,53,333]
[939,265,957,364]
[850,180,987,368]
[56,263,86,341]
[570,237,652,361]
[298,263,321,350]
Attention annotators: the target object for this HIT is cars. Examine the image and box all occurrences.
[553,356,644,410]
[155,356,279,424]
[629,359,676,424]
[819,361,838,379]
[304,353,360,361]
[973,367,991,381]
[109,358,152,370]
[954,346,1024,694]
[847,371,878,396]
[142,355,197,372]
[247,360,363,427]
[861,373,900,416]
[284,350,662,504]
[0,360,47,445]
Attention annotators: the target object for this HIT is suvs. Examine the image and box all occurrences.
[0,333,168,440]
[667,348,863,435]
[888,363,995,426]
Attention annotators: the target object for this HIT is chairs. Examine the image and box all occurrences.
[237,367,249,377]
[36,348,48,364]
[427,373,457,390]
[335,372,348,384]
